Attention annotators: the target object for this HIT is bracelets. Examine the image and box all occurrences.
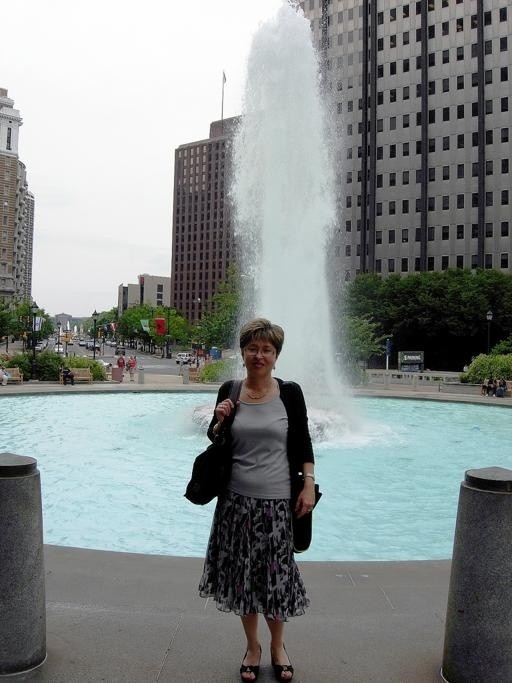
[303,472,315,479]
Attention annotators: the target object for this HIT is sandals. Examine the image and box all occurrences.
[270,642,293,682]
[240,645,261,683]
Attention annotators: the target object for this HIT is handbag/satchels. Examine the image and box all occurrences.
[184,444,227,506]
[290,477,322,553]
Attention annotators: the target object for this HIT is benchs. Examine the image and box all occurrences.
[58,366,94,386]
[482,379,512,397]
[0,366,24,385]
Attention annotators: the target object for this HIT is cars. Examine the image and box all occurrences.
[55,342,63,352]
[115,346,125,355]
[175,352,196,364]
[106,340,111,345]
[79,341,85,345]
[35,343,43,352]
[110,341,117,346]
[67,339,73,345]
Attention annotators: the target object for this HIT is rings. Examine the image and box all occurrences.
[307,507,313,512]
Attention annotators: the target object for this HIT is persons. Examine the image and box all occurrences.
[62,367,74,385]
[187,354,195,367]
[481,376,507,397]
[463,364,469,373]
[206,317,317,682]
[116,352,137,372]
[179,357,185,376]
[0,365,9,385]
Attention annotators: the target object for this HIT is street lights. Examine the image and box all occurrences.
[56,321,61,354]
[91,309,99,360]
[30,302,39,379]
[485,310,494,354]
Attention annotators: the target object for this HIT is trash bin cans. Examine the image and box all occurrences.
[210,350,222,358]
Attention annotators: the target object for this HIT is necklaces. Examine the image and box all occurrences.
[244,380,272,400]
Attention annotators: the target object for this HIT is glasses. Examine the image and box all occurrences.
[243,346,277,358]
